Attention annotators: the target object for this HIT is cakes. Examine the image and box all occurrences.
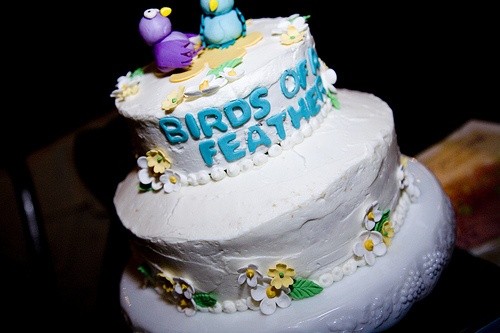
[109,0,457,331]
